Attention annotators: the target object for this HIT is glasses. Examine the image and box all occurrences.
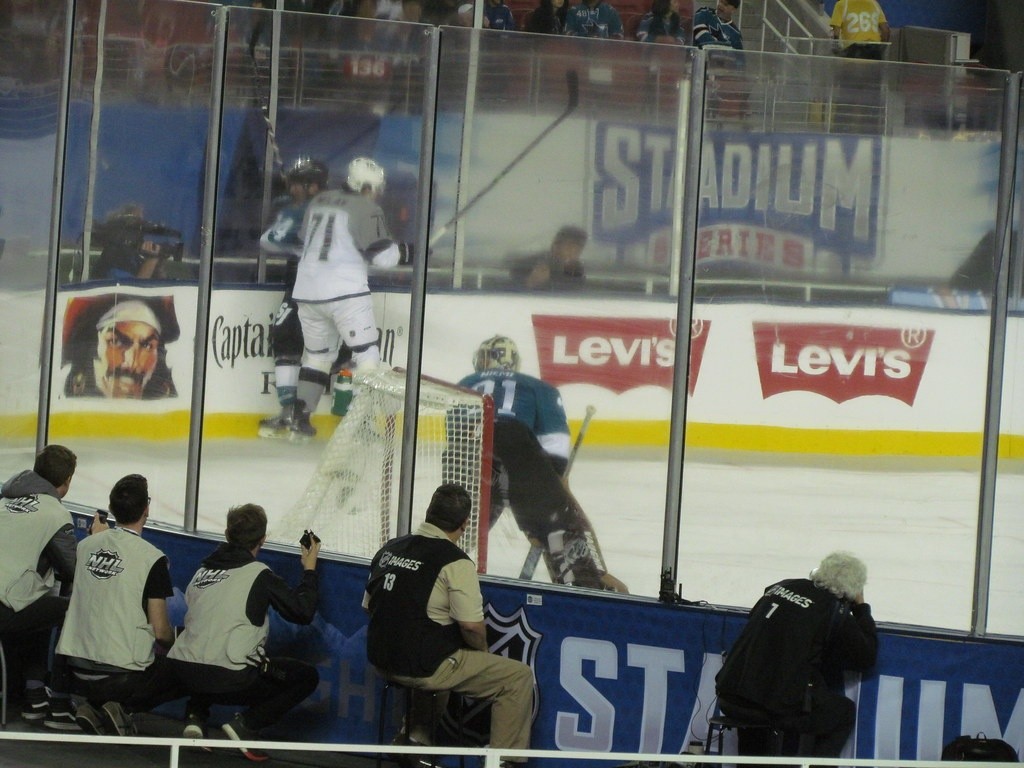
[146,497,151,504]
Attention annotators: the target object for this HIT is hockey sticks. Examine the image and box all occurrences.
[429,69,580,246]
[247,20,287,184]
[320,94,406,165]
[518,405,596,580]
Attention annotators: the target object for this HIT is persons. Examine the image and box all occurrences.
[165,504,320,761]
[289,159,413,444]
[257,156,356,440]
[515,226,590,288]
[92,201,172,280]
[717,552,880,768]
[0,445,110,729]
[830,0,890,133]
[524,0,624,41]
[693,0,744,51]
[363,486,533,768]
[53,475,176,735]
[638,0,691,47]
[447,336,621,593]
[245,0,514,48]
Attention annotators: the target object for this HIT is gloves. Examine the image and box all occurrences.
[399,242,432,266]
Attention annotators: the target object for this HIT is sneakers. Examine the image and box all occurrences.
[288,399,318,443]
[102,701,137,737]
[183,713,212,752]
[44,701,82,730]
[221,712,270,760]
[355,420,382,442]
[257,404,293,439]
[75,702,107,736]
[21,685,53,720]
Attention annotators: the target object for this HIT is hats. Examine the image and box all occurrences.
[726,0,740,9]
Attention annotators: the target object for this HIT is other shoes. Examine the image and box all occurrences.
[389,734,444,768]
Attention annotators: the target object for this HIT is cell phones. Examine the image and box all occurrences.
[88,509,109,535]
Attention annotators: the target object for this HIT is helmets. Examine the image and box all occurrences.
[289,159,328,187]
[347,157,384,193]
[472,336,521,373]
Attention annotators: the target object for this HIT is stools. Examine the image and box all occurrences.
[378,682,466,768]
[703,717,784,768]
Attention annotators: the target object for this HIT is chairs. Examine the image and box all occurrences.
[14,0,754,119]
